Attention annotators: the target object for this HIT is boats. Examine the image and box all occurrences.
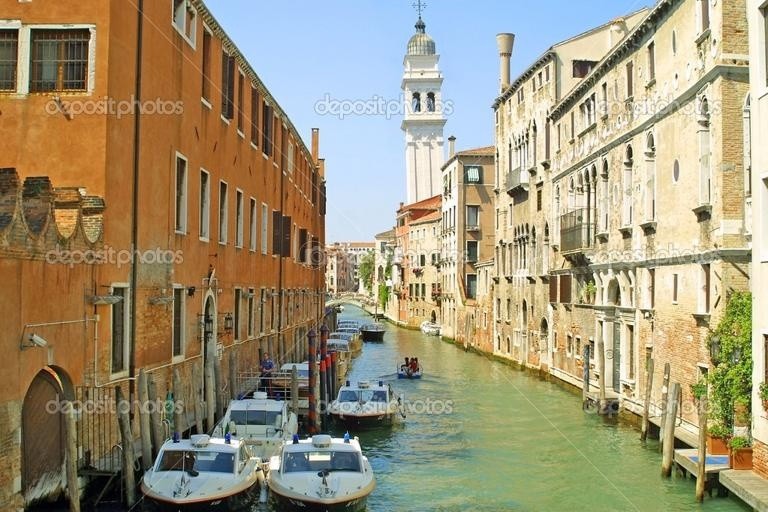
[420,321,441,335]
[397,362,422,379]
[140,320,393,509]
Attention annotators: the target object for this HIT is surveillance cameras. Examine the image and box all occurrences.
[27,333,48,349]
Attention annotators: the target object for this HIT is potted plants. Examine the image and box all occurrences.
[705,424,752,470]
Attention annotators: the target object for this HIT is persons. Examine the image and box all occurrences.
[401,357,409,368]
[406,357,416,373]
[259,352,275,398]
[414,357,419,371]
